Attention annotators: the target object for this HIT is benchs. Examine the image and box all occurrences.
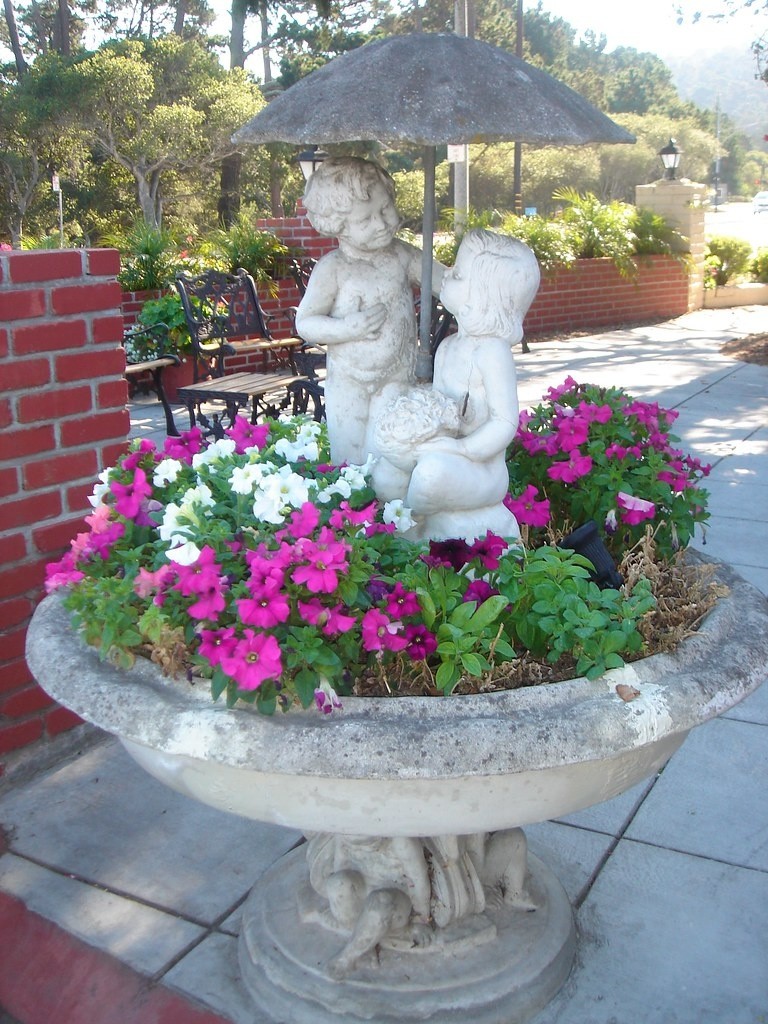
[122,322,180,437]
[292,295,458,423]
[175,267,304,385]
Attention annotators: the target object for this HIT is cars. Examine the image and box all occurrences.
[753,191,768,214]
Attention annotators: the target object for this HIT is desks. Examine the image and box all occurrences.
[177,372,308,444]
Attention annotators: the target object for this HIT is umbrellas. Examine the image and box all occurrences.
[230,33,637,377]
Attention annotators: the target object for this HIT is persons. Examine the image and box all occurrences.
[295,157,446,466]
[371,227,541,516]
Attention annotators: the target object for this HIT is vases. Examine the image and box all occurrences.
[25,546,768,1024]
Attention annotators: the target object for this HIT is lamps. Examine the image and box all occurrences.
[557,521,625,594]
[290,144,330,181]
[656,138,684,180]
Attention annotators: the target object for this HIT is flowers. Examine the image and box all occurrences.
[46,375,714,715]
